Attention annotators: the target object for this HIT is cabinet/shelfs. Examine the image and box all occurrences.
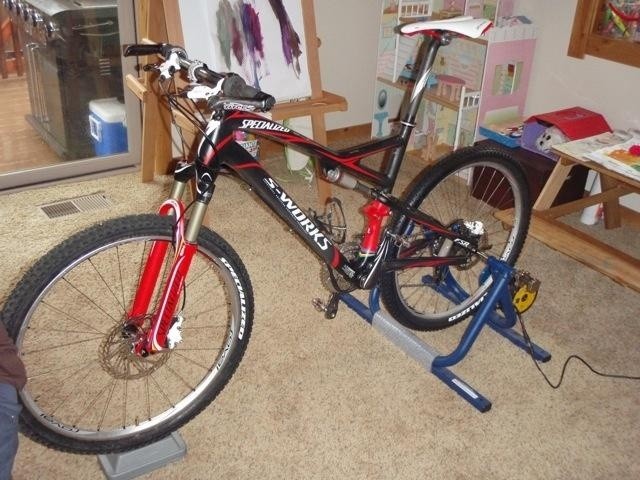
[368,1,535,186]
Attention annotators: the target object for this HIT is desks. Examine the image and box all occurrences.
[495,131,639,300]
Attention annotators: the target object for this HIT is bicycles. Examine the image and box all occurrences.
[1,15,532,455]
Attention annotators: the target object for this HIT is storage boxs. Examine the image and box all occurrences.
[87,97,128,157]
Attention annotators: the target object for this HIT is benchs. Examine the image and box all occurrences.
[496,206,639,302]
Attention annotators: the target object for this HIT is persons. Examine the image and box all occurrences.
[0,316,28,480]
